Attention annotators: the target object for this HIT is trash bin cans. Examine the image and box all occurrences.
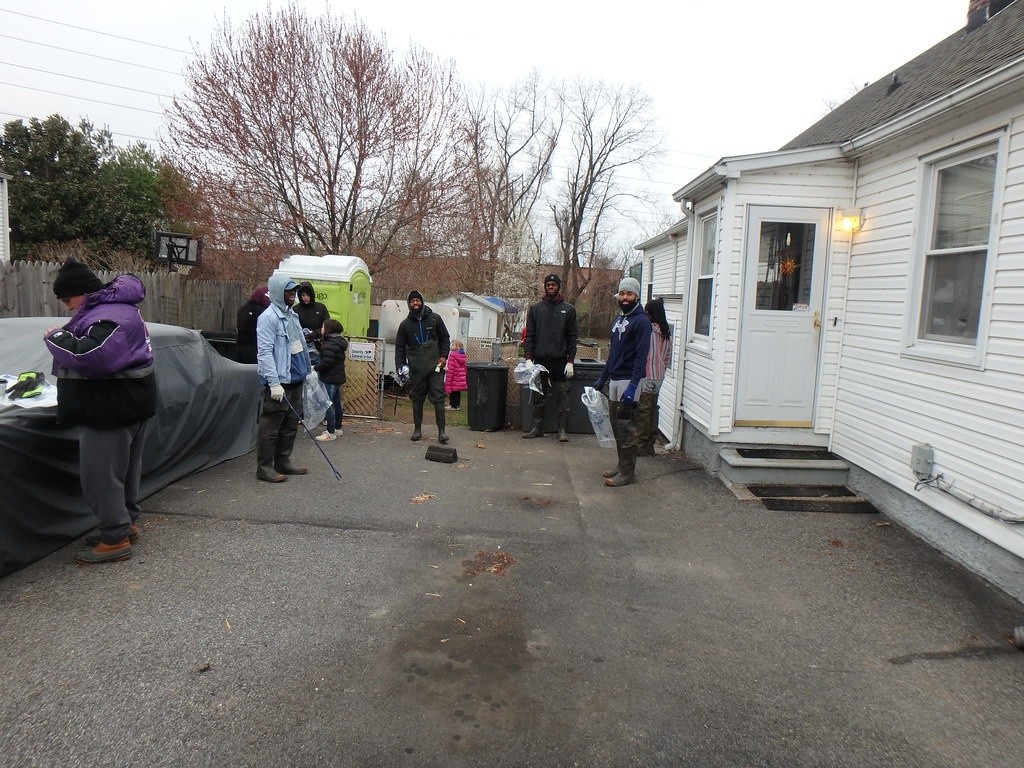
[465,363,510,432]
[520,358,609,434]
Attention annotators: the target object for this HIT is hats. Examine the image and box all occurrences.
[301,286,312,294]
[53,256,103,299]
[545,275,561,287]
[619,278,641,296]
[250,286,269,304]
[285,282,303,290]
[409,291,423,300]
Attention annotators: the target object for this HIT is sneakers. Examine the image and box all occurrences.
[80,520,139,546]
[316,431,337,441]
[445,405,456,411]
[73,536,132,565]
[323,421,328,425]
[323,428,343,435]
[456,406,462,410]
[299,420,304,424]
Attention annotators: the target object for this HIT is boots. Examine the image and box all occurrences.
[257,403,657,486]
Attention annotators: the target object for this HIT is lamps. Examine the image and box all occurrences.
[842,209,865,232]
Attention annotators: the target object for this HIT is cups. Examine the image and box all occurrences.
[0,378,8,402]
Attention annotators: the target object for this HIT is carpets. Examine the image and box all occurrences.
[763,498,878,511]
[748,483,856,497]
[735,446,840,460]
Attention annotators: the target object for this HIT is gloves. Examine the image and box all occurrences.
[396,367,407,383]
[303,327,311,336]
[593,378,605,391]
[23,373,45,398]
[620,384,637,407]
[564,363,574,379]
[525,359,534,369]
[6,372,37,400]
[270,383,286,402]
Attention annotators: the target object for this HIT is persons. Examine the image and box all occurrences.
[44,256,158,565]
[395,290,450,442]
[236,286,271,365]
[594,278,651,486]
[521,326,527,358]
[444,340,467,410]
[635,300,672,456]
[522,274,577,441]
[313,319,348,442]
[256,274,312,482]
[292,281,330,426]
